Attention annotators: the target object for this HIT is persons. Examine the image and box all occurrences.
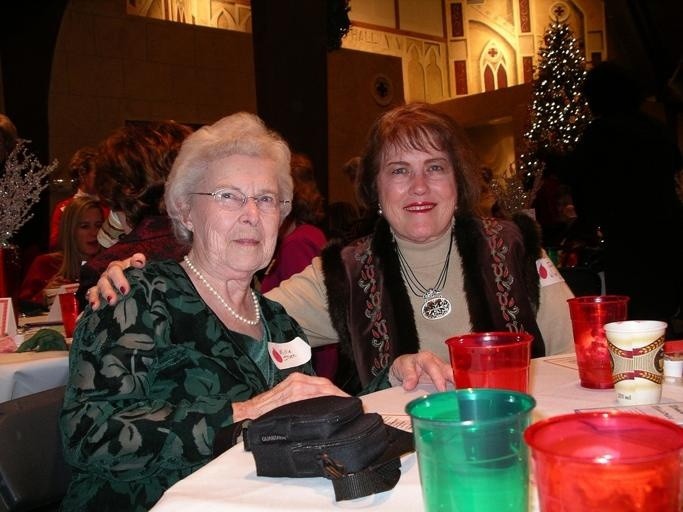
[55,111,454,507]
[87,99,593,399]
[463,131,605,296]
[250,153,378,381]
[0,113,194,315]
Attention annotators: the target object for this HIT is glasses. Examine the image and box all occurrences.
[196,189,290,214]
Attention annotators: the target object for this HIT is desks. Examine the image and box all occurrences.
[1,300,102,460]
[147,347,681,511]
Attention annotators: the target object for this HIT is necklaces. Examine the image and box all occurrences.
[184,256,261,325]
[389,224,452,321]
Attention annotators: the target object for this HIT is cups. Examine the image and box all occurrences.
[444,332,534,469]
[58,292,82,339]
[603,319,667,406]
[523,409,682,511]
[0,298,9,339]
[565,295,629,391]
[405,385,535,512]
[95,211,131,249]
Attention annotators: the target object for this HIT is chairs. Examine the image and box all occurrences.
[0,399,69,512]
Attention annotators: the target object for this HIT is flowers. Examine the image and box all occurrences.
[0,134,60,252]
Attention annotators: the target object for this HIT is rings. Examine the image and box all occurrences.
[81,288,96,299]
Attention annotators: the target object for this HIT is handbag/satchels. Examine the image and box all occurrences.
[244,394,415,500]
